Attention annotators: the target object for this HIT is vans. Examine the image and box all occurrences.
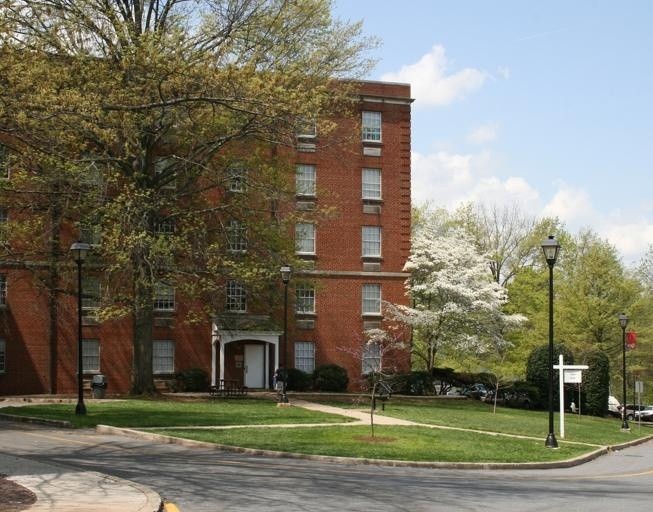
[570,395,653,421]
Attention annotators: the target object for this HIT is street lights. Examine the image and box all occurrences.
[541,236,561,448]
[69,242,91,414]
[618,313,631,429]
[279,265,293,403]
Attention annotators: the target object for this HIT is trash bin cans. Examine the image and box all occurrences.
[92,375,106,398]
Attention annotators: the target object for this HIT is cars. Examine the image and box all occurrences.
[411,382,533,410]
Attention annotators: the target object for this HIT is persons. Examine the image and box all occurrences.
[273,359,289,402]
